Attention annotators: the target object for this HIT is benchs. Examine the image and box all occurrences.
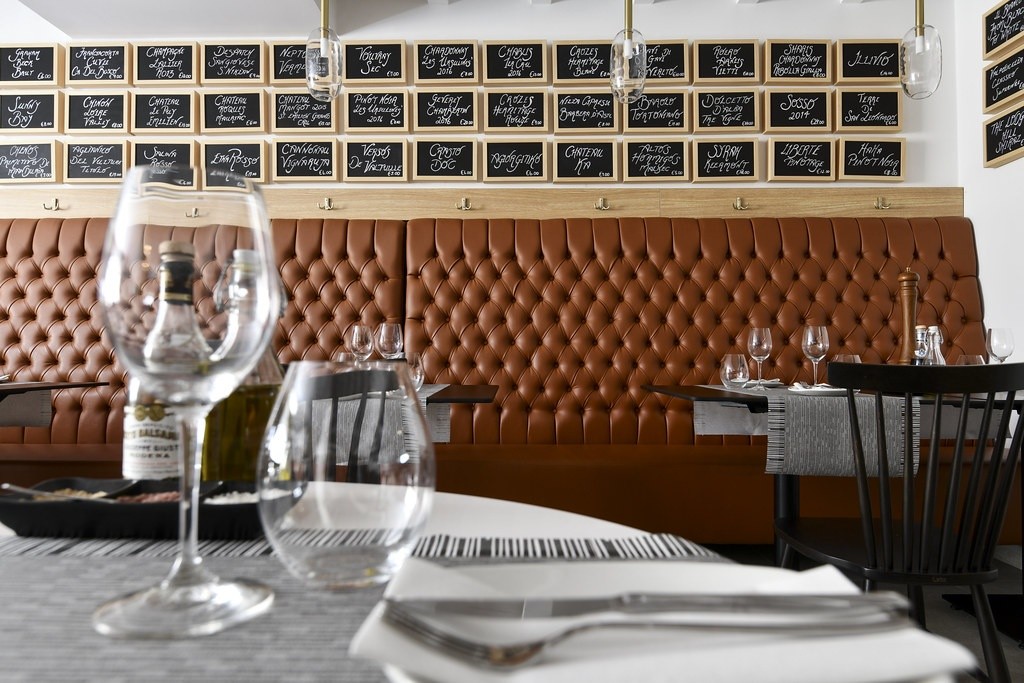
[0,219,1024,548]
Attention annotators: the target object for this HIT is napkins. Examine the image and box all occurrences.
[346,552,975,680]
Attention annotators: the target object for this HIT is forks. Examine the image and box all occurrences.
[381,599,900,675]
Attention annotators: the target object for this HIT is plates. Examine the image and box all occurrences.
[731,380,784,388]
[0,468,377,541]
[788,385,860,397]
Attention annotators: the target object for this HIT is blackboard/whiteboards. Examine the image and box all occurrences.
[979,0,1024,171]
[0,35,909,184]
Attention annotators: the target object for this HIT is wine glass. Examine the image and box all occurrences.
[801,326,829,387]
[986,327,1015,364]
[747,327,773,394]
[89,162,282,644]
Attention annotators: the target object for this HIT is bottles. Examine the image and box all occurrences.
[911,325,927,366]
[921,326,946,366]
[121,240,211,484]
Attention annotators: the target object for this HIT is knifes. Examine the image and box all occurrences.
[397,588,909,621]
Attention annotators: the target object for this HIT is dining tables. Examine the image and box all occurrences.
[0,477,963,683]
[262,379,500,467]
[638,383,1024,645]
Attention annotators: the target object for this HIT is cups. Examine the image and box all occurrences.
[404,352,425,393]
[254,361,436,592]
[336,352,355,367]
[955,354,985,365]
[719,353,750,391]
[831,354,862,363]
[375,322,404,361]
[350,324,375,363]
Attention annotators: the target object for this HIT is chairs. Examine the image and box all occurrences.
[827,360,1024,683]
[290,370,398,488]
